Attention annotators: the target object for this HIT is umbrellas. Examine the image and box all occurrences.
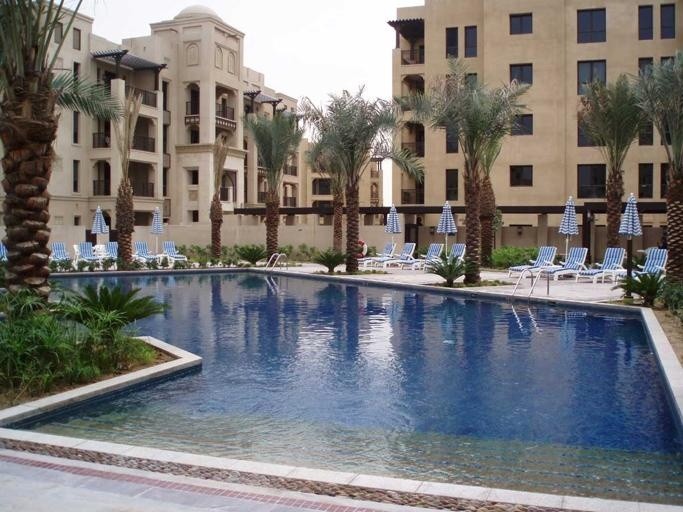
[148,206,164,255]
[435,201,459,259]
[90,205,109,258]
[555,195,581,266]
[384,203,403,260]
[617,194,643,241]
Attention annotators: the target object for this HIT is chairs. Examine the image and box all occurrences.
[50,242,187,269]
[358,242,466,274]
[0,241,7,258]
[508,246,667,286]
